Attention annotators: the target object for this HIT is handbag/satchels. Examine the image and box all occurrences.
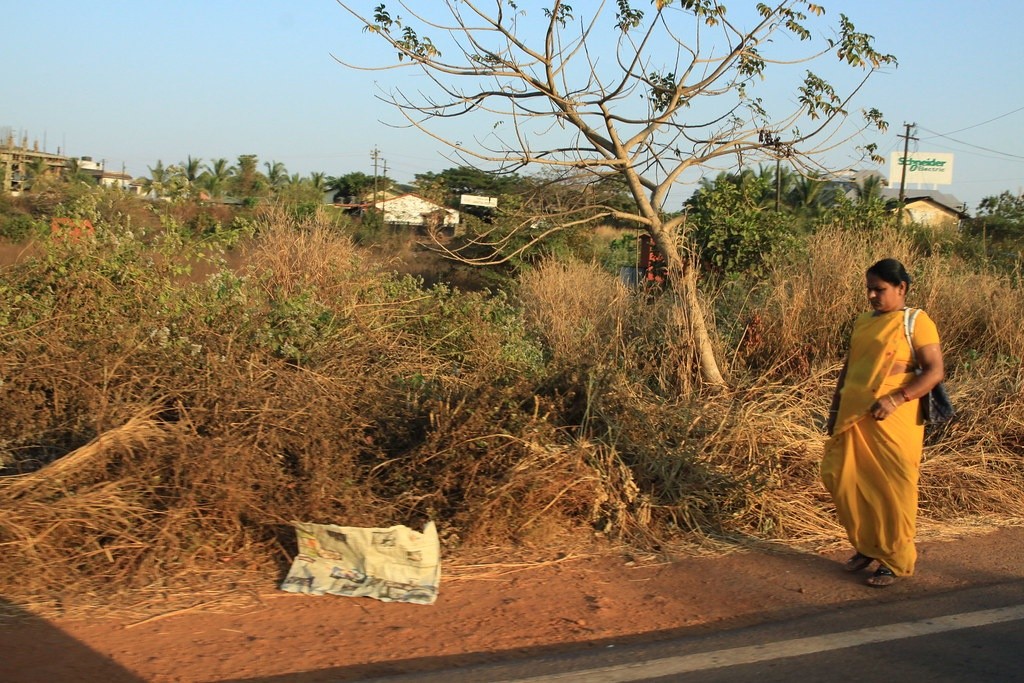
[904,308,955,424]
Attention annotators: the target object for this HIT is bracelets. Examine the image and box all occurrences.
[828,409,838,413]
[887,392,897,408]
[899,386,910,402]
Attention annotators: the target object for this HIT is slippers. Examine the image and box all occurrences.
[844,552,874,572]
[867,569,904,587]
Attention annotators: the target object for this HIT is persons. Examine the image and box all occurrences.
[821,257,944,587]
